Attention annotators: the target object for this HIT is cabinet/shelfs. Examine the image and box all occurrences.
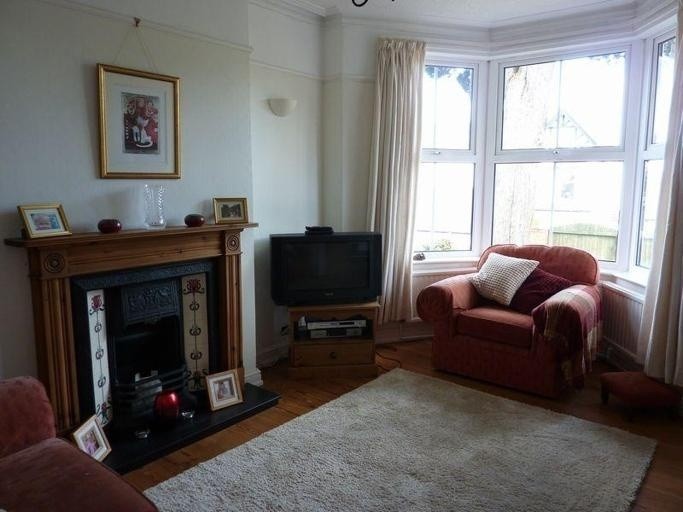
[4,221,260,432]
[289,302,382,382]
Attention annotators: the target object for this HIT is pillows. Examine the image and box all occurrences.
[510,268,573,317]
[468,251,540,307]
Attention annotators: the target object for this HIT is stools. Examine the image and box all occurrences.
[599,372,676,420]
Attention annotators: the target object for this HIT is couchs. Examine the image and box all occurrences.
[414,244,602,401]
[1,376,160,512]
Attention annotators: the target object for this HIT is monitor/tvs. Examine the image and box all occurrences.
[269,232,382,306]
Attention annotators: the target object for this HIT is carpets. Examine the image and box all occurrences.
[142,365,657,512]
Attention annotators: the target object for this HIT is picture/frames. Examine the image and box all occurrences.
[205,369,243,412]
[213,198,249,224]
[71,414,111,463]
[17,203,72,239]
[96,62,181,179]
